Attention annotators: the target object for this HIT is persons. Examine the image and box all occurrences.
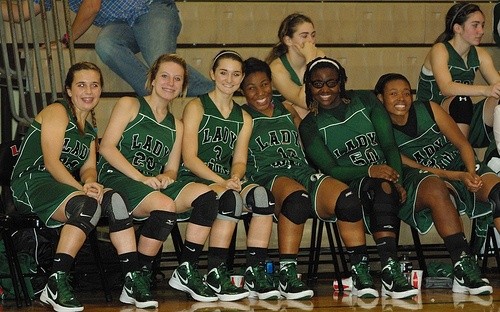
[264,14,322,118]
[238,58,379,301]
[377,72,500,296]
[95,54,219,300]
[417,3,500,147]
[8,63,159,312]
[301,58,418,299]
[177,50,281,301]
[0,0,215,98]
[475,105,500,178]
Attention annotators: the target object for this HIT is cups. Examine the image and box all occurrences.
[410,270,423,292]
[332,290,357,306]
[229,275,244,289]
[333,276,353,292]
[412,293,424,310]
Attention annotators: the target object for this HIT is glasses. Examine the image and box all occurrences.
[310,77,340,88]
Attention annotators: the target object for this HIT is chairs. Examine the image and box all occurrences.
[0,137,500,308]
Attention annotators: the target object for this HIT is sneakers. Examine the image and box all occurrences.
[451,255,493,295]
[277,262,314,299]
[119,272,159,308]
[168,260,218,302]
[351,256,379,297]
[202,265,249,302]
[381,256,418,299]
[141,264,154,285]
[243,266,281,300]
[39,270,85,312]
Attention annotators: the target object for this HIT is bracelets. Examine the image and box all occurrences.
[62,32,69,48]
